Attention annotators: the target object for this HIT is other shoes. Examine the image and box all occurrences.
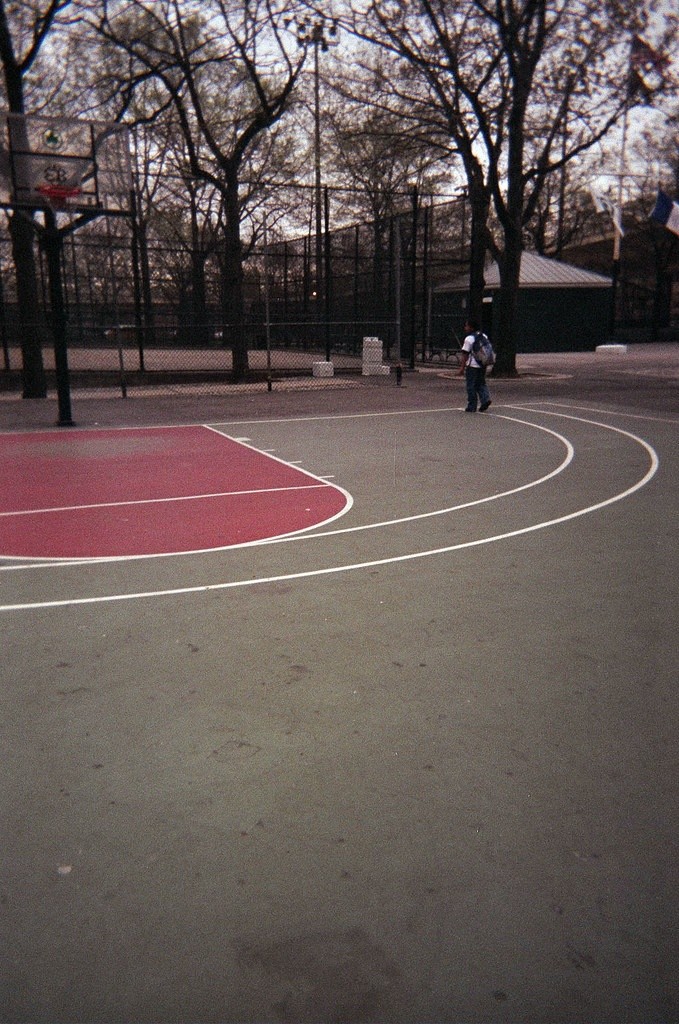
[465,407,476,412]
[480,400,492,412]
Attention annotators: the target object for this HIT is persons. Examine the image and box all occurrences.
[458,319,495,413]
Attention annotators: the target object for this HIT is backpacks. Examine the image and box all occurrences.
[473,331,496,366]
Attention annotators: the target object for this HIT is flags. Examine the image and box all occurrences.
[650,187,679,235]
[590,186,624,238]
